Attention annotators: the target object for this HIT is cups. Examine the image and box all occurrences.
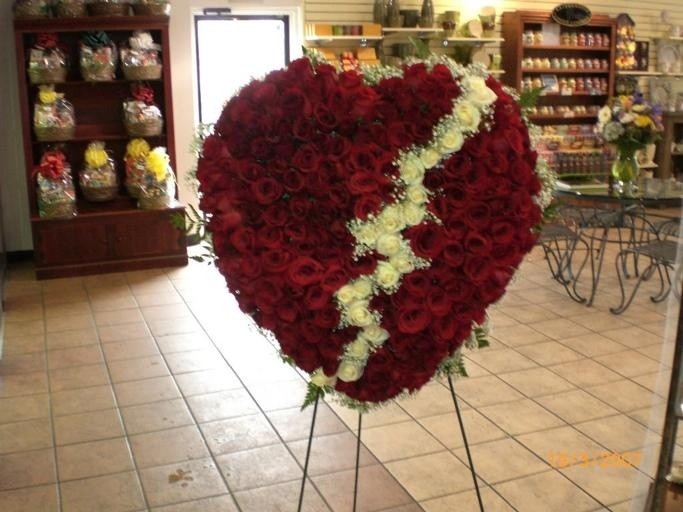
[389,15,405,27]
[460,19,483,37]
[400,9,419,27]
[333,25,362,35]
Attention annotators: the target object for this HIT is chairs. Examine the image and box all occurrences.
[538,194,683,315]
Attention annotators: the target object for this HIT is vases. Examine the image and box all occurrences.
[613,146,640,197]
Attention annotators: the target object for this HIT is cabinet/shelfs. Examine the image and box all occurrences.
[618,35,683,78]
[11,13,189,280]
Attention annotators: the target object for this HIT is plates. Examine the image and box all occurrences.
[657,44,680,65]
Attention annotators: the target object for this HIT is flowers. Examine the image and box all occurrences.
[173,47,557,412]
[595,94,666,143]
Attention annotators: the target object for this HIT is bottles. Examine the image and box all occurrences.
[438,12,456,38]
[479,5,496,38]
[545,152,605,174]
[523,57,608,69]
[522,76,608,91]
[420,0,435,28]
[373,0,389,27]
[525,105,600,116]
[524,31,610,48]
[390,0,400,17]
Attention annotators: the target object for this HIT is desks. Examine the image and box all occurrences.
[552,185,683,307]
[655,109,682,181]
